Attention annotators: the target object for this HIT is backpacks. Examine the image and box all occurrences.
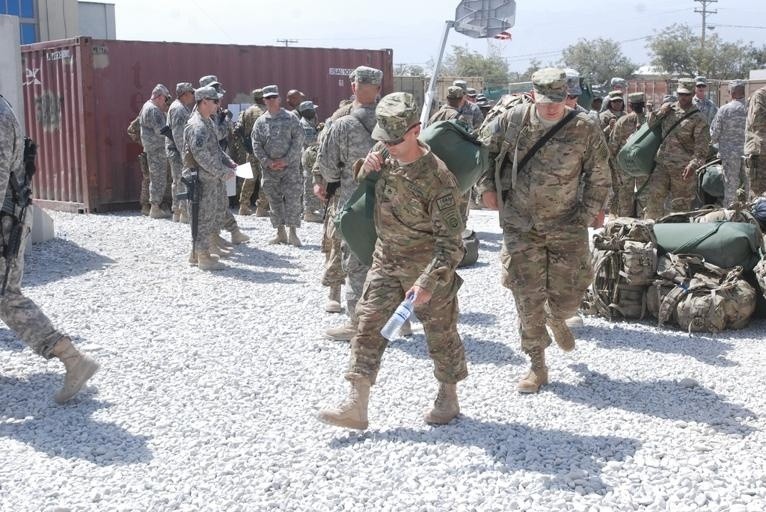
[579,193,766,339]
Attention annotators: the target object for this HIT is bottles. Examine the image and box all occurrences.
[379,295,417,340]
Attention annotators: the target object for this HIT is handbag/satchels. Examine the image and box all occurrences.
[616,123,662,177]
[701,143,725,197]
[334,122,489,269]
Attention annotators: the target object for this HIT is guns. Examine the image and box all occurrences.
[181,168,202,259]
[0,137,37,295]
[160,126,177,151]
[323,182,341,220]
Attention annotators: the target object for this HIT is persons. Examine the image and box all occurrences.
[127,75,350,269]
[474,67,613,395]
[564,68,613,328]
[743,87,765,201]
[429,80,485,228]
[592,67,677,219]
[692,76,719,124]
[314,91,468,430]
[0,92,100,404]
[313,66,411,340]
[643,78,711,220]
[709,80,748,210]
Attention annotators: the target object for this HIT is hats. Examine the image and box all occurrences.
[593,77,746,100]
[350,66,582,143]
[150,76,319,114]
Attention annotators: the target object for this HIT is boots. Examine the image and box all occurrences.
[140,202,325,272]
[317,283,574,430]
[51,337,101,403]
